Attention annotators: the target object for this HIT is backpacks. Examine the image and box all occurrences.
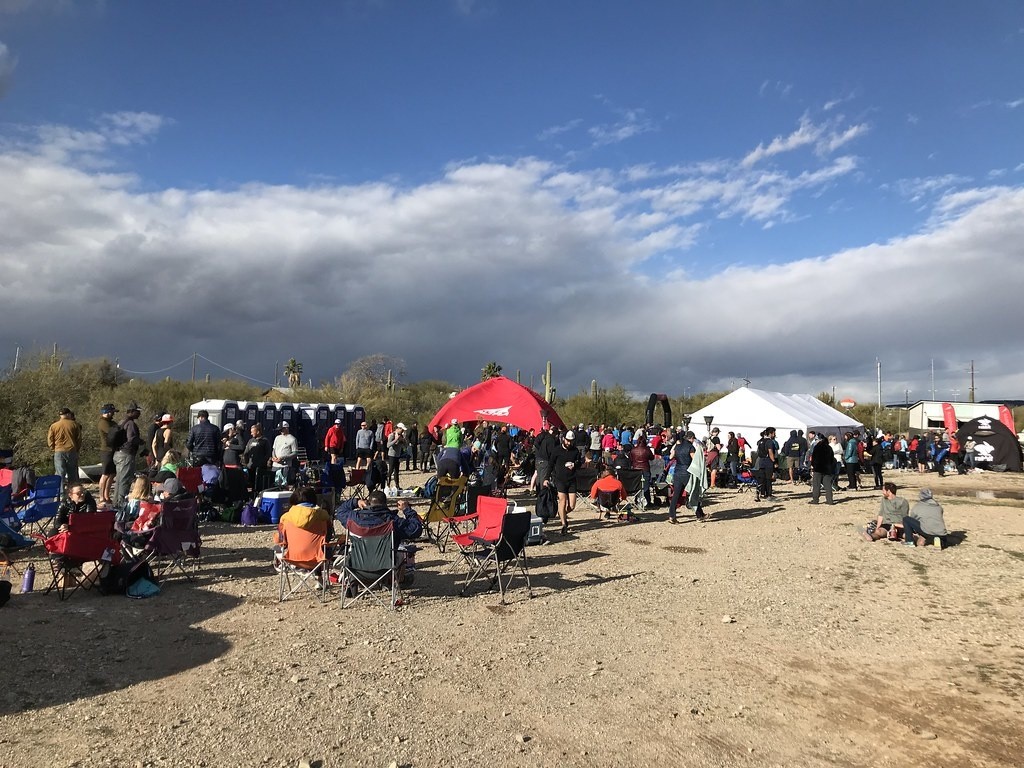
[222,506,242,523]
[106,425,127,449]
[240,503,257,526]
[207,508,222,522]
[424,476,439,498]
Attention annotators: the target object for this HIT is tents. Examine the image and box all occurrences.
[427,376,568,442]
[954,415,1023,473]
[683,387,865,463]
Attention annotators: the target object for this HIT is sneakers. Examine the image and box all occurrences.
[900,541,915,548]
[933,537,942,551]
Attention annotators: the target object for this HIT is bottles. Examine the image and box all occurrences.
[253,496,261,507]
[22,562,36,592]
[4,569,10,582]
[383,485,398,496]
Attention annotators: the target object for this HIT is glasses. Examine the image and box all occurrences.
[71,488,88,496]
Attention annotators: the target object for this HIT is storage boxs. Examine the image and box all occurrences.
[262,490,296,524]
[525,513,543,546]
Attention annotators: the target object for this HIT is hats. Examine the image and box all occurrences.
[221,422,234,433]
[274,421,290,431]
[564,431,576,441]
[101,404,120,412]
[149,469,176,483]
[686,431,697,439]
[58,407,71,415]
[196,411,209,419]
[712,428,721,433]
[369,492,387,504]
[157,478,182,494]
[334,418,461,432]
[127,401,144,411]
[160,414,174,422]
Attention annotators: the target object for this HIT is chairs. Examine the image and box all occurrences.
[819,461,842,493]
[717,450,758,494]
[576,455,686,524]
[274,447,531,611]
[0,466,205,603]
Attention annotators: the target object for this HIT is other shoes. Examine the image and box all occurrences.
[315,581,324,591]
[406,465,436,474]
[555,463,994,535]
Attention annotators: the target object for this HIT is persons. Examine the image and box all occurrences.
[868,482,908,546]
[418,417,975,535]
[903,489,949,550]
[43,404,418,596]
